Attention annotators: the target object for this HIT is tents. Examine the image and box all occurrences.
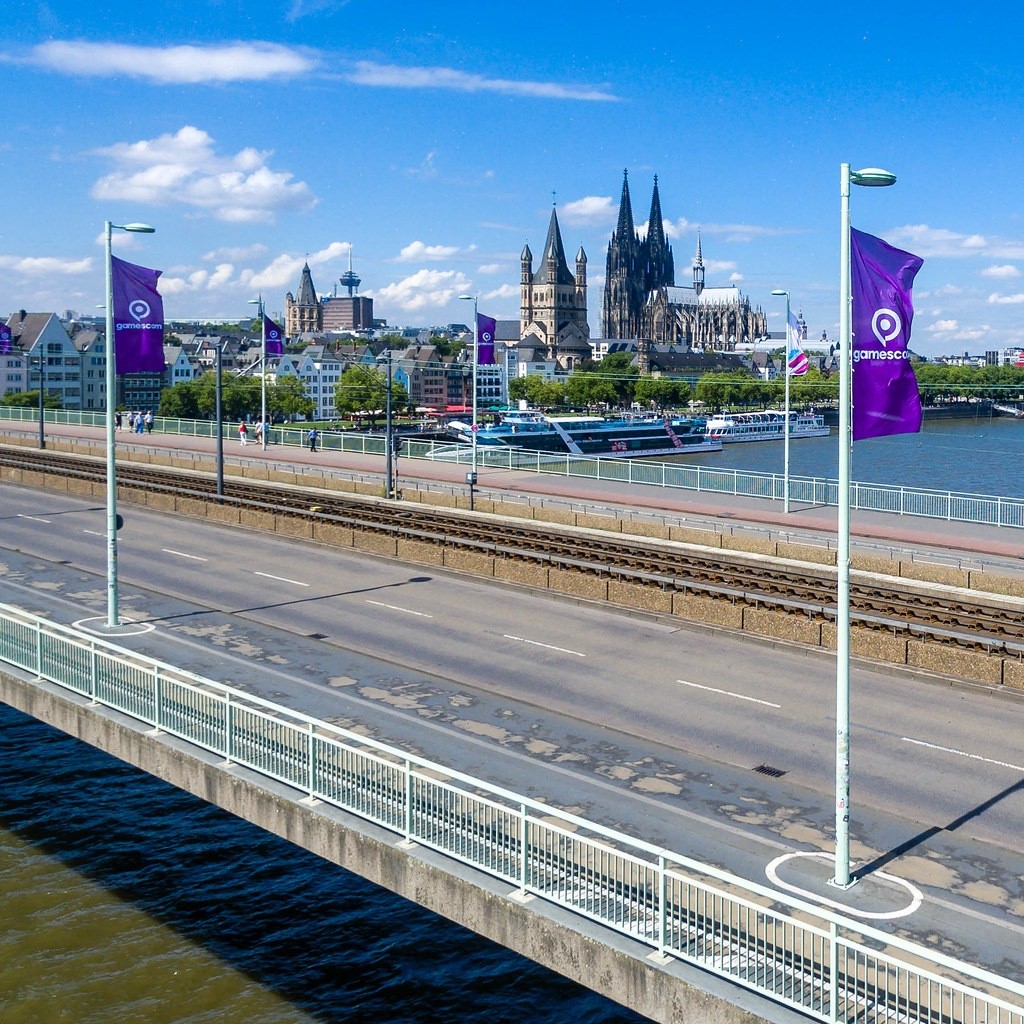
[483,406,518,411]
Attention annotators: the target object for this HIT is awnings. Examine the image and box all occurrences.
[351,406,438,413]
[446,406,472,411]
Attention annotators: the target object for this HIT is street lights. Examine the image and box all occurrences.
[104,221,155,627]
[771,290,789,514]
[247,299,266,452]
[458,294,478,473]
[833,155,899,891]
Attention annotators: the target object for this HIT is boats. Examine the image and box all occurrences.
[698,410,832,444]
[446,407,724,461]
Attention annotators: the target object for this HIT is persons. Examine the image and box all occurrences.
[579,397,836,431]
[254,419,270,446]
[237,421,247,446]
[461,412,549,438]
[306,426,321,452]
[127,410,136,433]
[919,397,1022,415]
[348,418,452,436]
[332,422,336,430]
[134,412,145,437]
[114,409,123,433]
[145,411,155,435]
